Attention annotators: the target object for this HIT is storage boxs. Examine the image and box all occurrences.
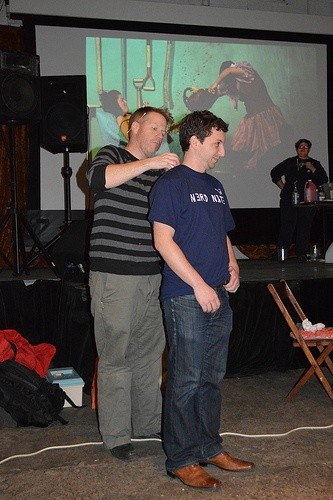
[47,367,85,408]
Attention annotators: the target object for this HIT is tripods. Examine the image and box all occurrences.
[22,154,90,274]
[0,123,58,280]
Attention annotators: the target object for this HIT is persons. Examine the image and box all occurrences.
[100,90,132,141]
[85,107,181,460]
[208,61,285,168]
[270,139,329,264]
[147,110,254,489]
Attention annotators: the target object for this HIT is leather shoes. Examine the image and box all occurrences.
[199,450,255,472]
[167,463,223,492]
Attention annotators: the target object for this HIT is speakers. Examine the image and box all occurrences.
[38,75,88,154]
[0,51,42,123]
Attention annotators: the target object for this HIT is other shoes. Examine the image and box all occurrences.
[110,443,137,461]
[130,432,162,440]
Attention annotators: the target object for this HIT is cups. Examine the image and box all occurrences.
[310,243,323,262]
[277,248,288,262]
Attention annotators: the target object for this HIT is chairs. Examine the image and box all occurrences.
[267,279,333,402]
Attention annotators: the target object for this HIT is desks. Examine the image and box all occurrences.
[293,200,333,260]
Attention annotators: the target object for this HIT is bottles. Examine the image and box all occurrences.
[304,180,317,204]
[292,186,300,205]
[318,185,326,202]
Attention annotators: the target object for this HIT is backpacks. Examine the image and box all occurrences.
[0,342,88,428]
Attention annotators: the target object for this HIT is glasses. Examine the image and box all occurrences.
[299,145,309,149]
